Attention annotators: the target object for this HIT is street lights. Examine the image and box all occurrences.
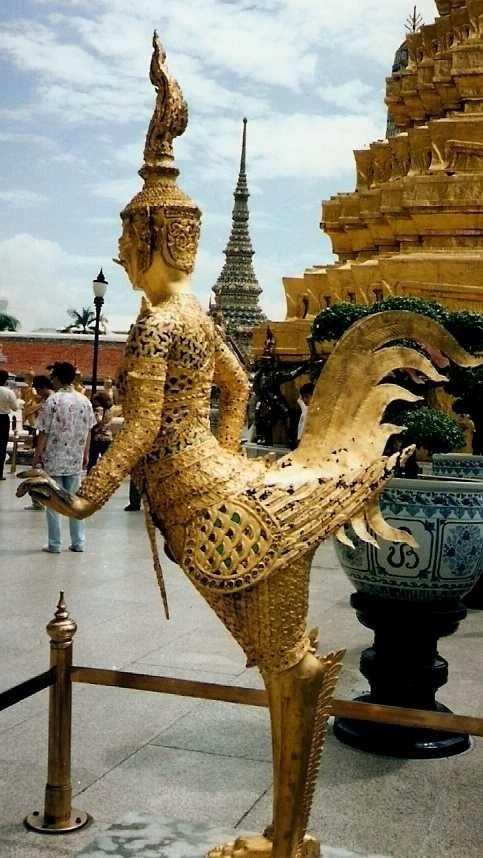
[91,267,109,412]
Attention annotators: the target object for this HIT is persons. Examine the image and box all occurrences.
[296,382,315,446]
[0,361,141,553]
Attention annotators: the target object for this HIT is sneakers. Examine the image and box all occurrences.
[68,544,84,552]
[42,544,61,553]
[23,504,44,510]
[124,504,140,511]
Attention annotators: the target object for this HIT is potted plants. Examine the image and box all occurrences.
[306,293,483,761]
